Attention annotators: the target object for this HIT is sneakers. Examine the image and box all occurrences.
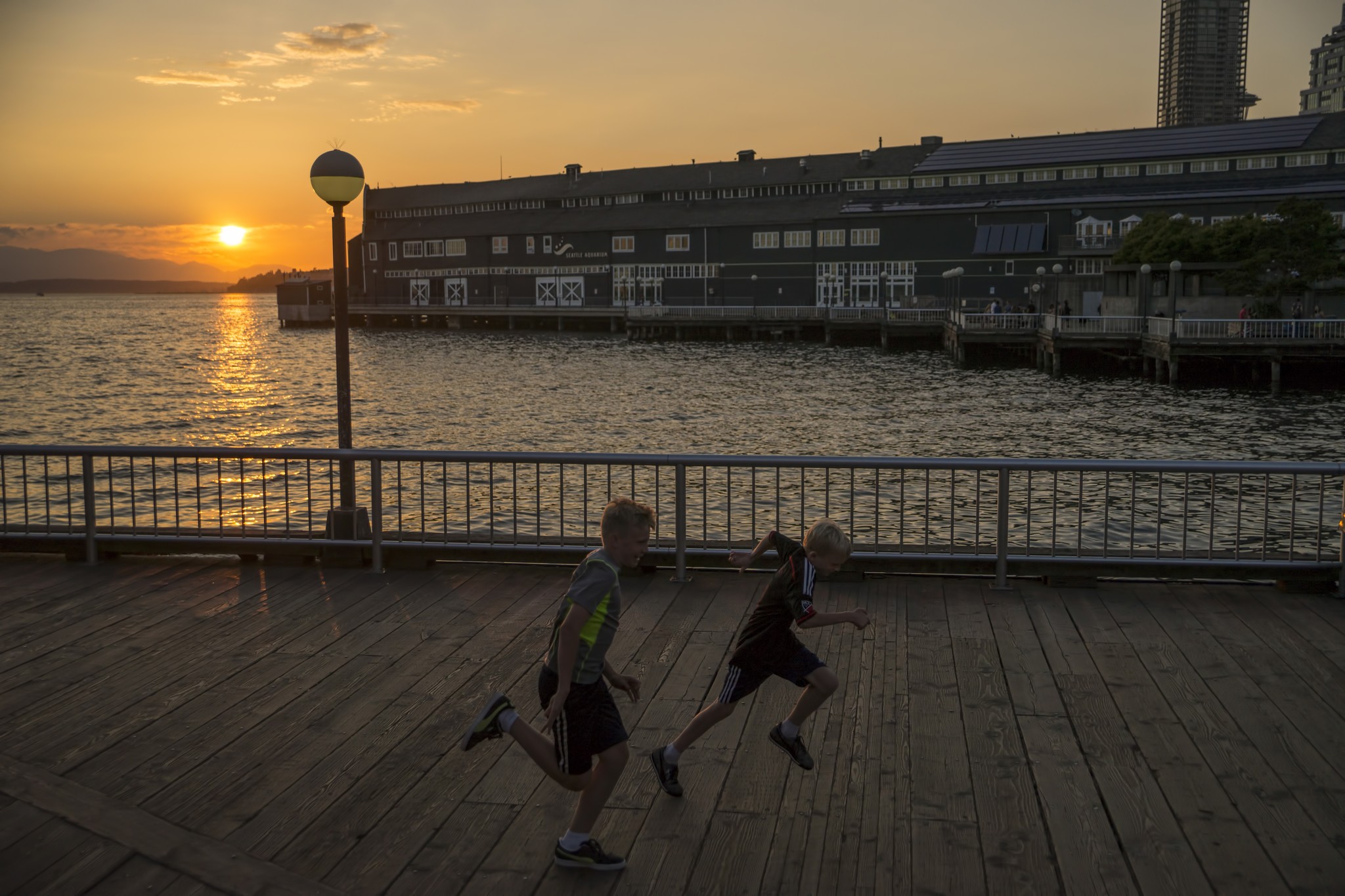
[554,837,626,872]
[768,721,815,770]
[650,745,683,797]
[460,691,515,753]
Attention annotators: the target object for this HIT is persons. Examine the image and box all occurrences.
[988,299,1073,329]
[1238,298,1326,338]
[460,497,657,871]
[650,517,871,797]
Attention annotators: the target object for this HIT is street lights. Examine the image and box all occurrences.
[504,267,510,307]
[649,277,665,306]
[823,273,836,321]
[721,263,726,306]
[942,266,965,331]
[1167,260,1182,344]
[458,268,462,307]
[373,269,377,306]
[1051,264,1063,339]
[283,272,311,283]
[636,276,642,306]
[1140,264,1151,333]
[621,276,628,320]
[415,268,419,307]
[310,149,371,540]
[606,265,611,308]
[1035,267,1046,330]
[553,266,558,307]
[751,275,758,319]
[880,271,887,326]
